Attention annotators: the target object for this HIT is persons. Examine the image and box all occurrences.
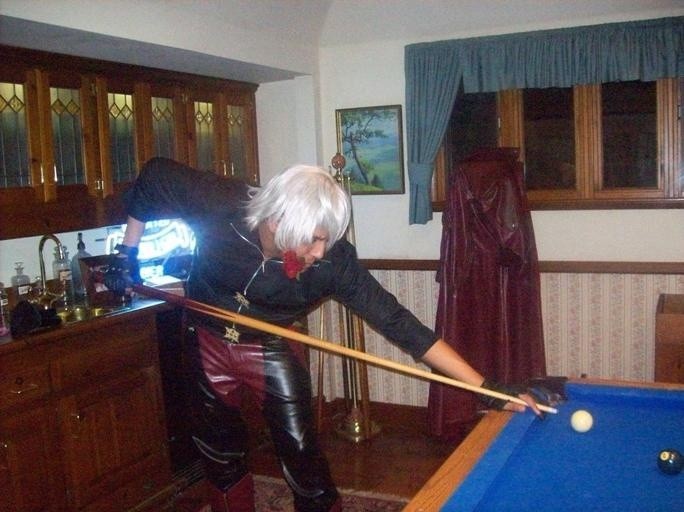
[105,158,548,512]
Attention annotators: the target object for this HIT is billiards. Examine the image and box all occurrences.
[657,446,683,477]
[570,409,595,433]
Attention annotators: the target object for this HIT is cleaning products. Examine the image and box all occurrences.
[70,231,94,299]
[11,260,34,303]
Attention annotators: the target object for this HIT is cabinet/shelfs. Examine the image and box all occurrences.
[1,43,262,242]
[0,268,309,511]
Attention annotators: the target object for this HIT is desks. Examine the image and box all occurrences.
[396,375,683,512]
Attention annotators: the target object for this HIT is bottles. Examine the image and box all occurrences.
[53,247,73,297]
[0,282,9,338]
[12,261,31,307]
[70,243,94,295]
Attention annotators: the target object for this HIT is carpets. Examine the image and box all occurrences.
[182,468,409,512]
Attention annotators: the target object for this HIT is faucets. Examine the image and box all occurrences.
[38,232,64,295]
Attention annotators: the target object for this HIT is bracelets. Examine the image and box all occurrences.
[120,244,138,256]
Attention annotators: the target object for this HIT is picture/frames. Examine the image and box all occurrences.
[79,253,144,305]
[332,101,406,198]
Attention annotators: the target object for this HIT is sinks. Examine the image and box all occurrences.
[55,304,133,324]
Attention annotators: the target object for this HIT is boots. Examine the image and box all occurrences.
[204,471,256,512]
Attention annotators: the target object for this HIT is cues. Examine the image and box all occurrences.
[346,174,372,448]
[337,297,351,415]
[89,270,560,416]
[316,300,326,440]
